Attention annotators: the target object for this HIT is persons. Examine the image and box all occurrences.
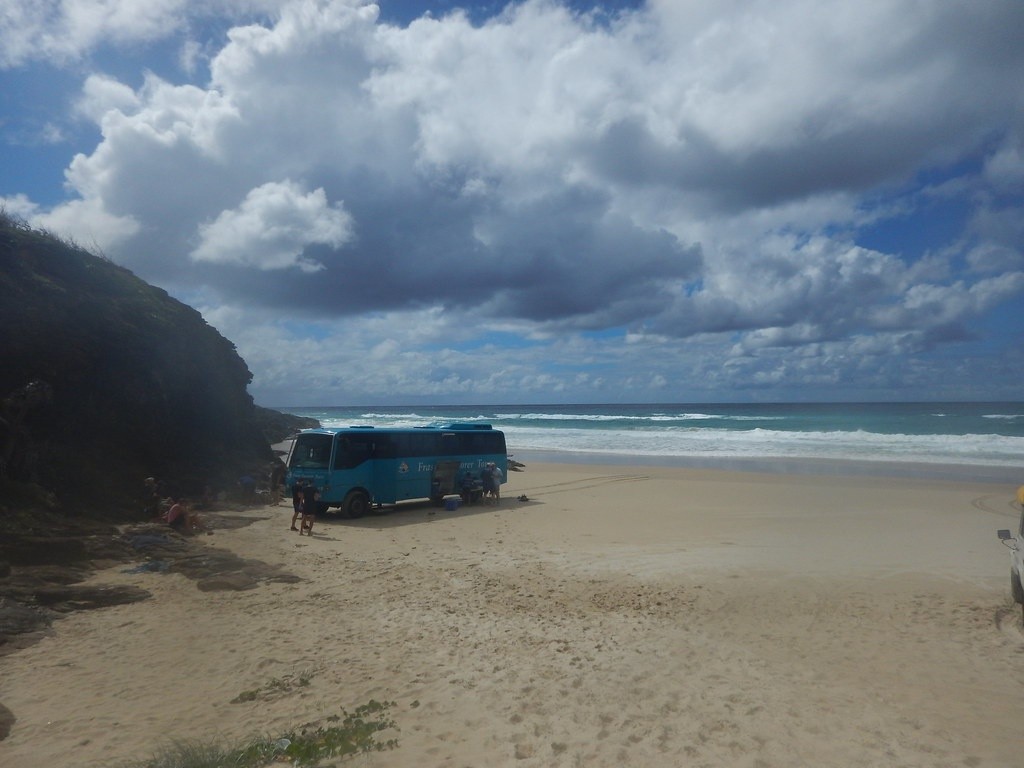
[239,467,258,509]
[267,466,282,507]
[143,476,213,537]
[462,471,477,506]
[480,462,504,507]
[290,478,320,536]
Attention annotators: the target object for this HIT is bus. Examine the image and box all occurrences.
[284,420,508,520]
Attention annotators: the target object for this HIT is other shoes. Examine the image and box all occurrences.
[304,525,310,529]
[291,527,299,531]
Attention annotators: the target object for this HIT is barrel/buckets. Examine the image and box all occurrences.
[446,500,459,511]
